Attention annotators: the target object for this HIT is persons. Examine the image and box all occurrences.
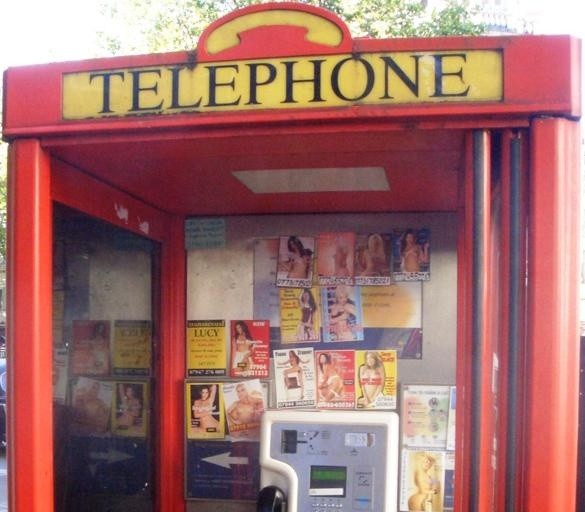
[232,322,268,380]
[275,351,388,411]
[73,383,107,429]
[408,452,441,512]
[117,383,141,429]
[276,228,430,342]
[192,382,264,441]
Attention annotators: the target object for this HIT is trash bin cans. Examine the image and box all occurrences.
[256,407,399,512]
